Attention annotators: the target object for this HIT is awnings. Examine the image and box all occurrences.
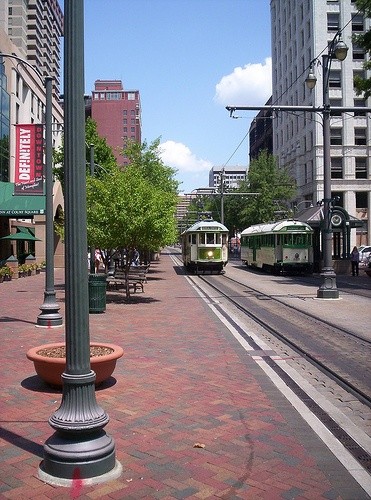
[19,227,35,236]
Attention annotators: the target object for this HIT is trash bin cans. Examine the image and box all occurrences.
[88,274,108,313]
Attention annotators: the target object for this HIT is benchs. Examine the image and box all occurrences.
[106,265,150,293]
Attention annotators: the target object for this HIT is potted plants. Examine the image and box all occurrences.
[0,265,14,283]
[17,261,46,278]
[27,342,125,387]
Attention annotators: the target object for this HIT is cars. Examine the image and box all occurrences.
[357,245,371,262]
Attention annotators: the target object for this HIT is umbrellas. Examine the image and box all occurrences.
[1,233,42,260]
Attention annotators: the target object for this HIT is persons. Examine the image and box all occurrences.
[95,249,140,270]
[350,246,360,276]
[233,243,238,257]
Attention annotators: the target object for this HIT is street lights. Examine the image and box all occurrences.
[315,30,353,301]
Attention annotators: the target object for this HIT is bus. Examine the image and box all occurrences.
[180,212,229,273]
[239,218,314,275]
